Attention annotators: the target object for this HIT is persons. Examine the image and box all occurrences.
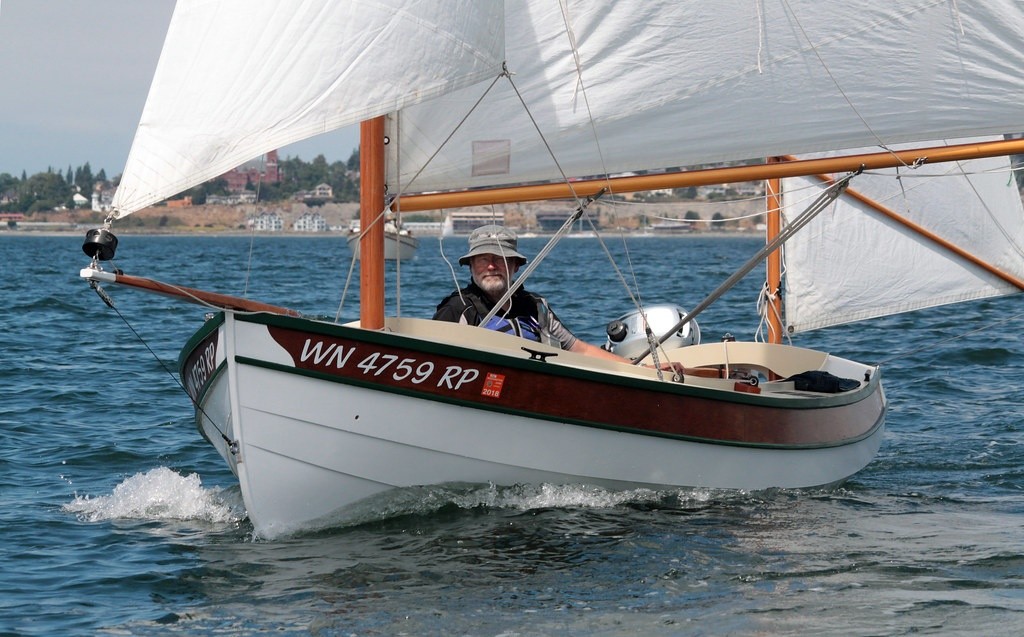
[433,224,687,374]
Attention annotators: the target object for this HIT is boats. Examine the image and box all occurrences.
[345,216,418,264]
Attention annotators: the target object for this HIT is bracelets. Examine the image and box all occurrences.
[638,363,646,367]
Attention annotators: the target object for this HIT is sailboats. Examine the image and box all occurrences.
[80,1,1024,537]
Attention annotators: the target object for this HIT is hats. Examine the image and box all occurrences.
[460,225,527,268]
